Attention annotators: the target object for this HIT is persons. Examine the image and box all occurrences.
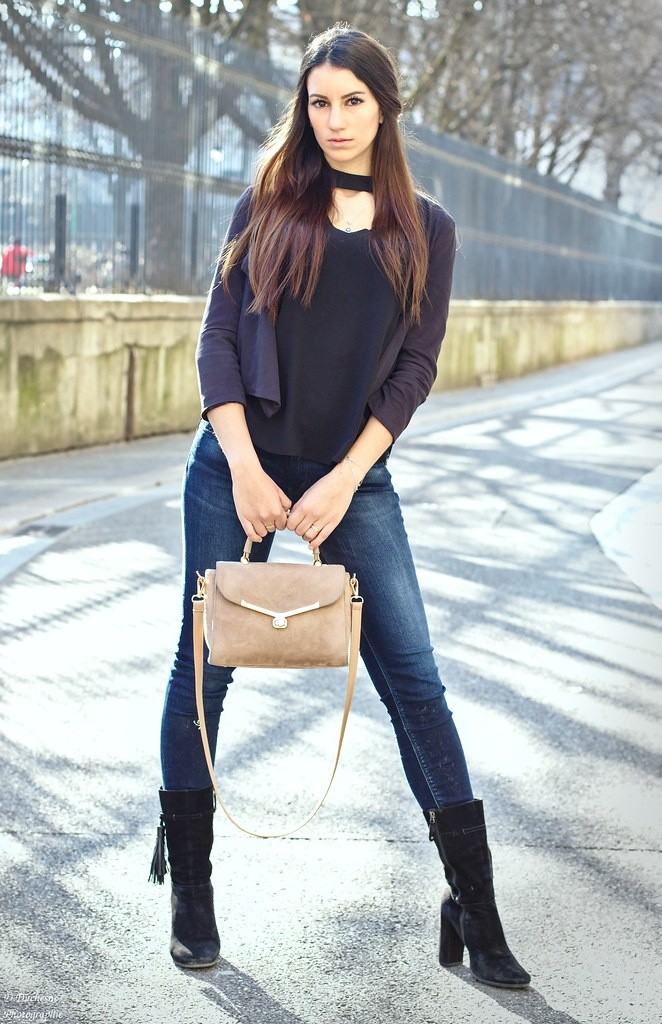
[159,26,533,991]
[4,238,27,285]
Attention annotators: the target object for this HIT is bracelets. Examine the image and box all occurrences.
[335,456,366,491]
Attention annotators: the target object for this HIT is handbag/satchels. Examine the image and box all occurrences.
[189,512,364,840]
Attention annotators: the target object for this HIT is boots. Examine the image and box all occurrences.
[153,785,222,968]
[423,798,533,988]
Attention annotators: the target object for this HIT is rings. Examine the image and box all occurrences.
[265,524,274,529]
[311,525,319,533]
